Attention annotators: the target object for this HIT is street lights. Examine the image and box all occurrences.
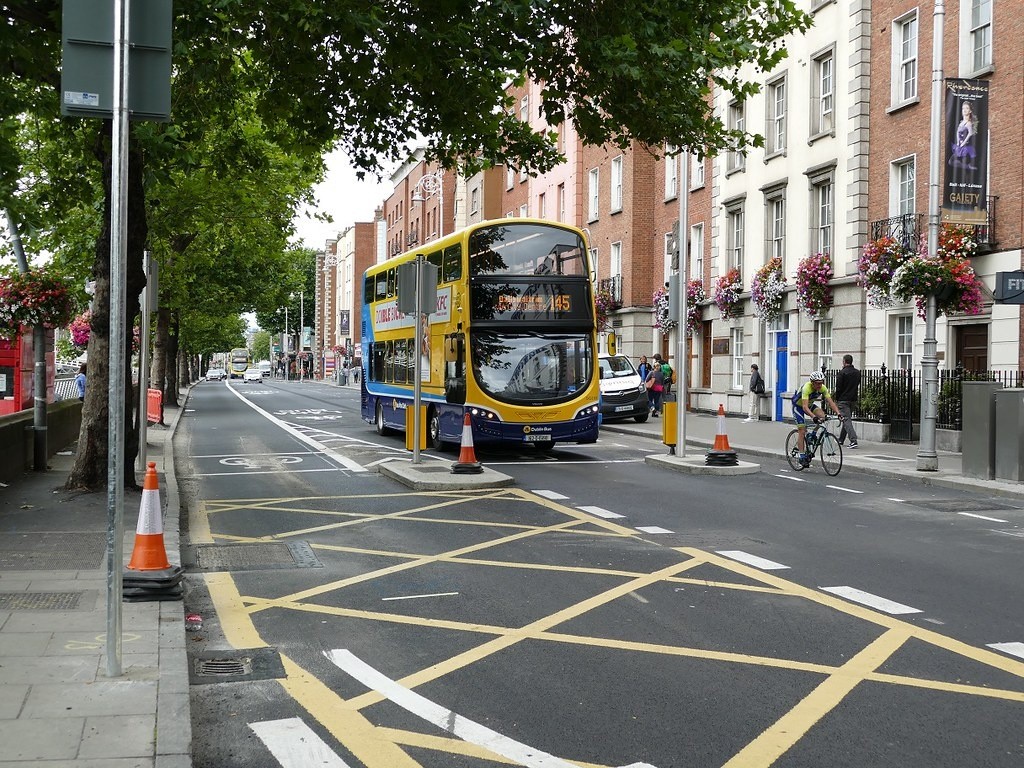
[410,161,445,239]
[275,307,288,381]
[289,291,305,383]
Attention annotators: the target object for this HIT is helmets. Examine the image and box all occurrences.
[810,371,825,383]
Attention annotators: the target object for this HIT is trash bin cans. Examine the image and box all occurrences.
[338,370,346,386]
[660,393,677,455]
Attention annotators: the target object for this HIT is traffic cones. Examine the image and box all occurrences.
[120,462,185,603]
[450,412,486,476]
[703,404,741,468]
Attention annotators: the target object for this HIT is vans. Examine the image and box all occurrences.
[255,360,271,377]
[596,352,652,423]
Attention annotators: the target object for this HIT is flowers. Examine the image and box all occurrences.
[132,326,141,353]
[69,307,92,350]
[715,267,744,321]
[749,256,787,326]
[686,277,705,335]
[652,286,678,337]
[1,264,72,349]
[792,254,833,320]
[330,344,348,357]
[855,219,987,322]
[593,285,616,335]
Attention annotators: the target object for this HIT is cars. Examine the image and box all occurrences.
[218,369,228,380]
[204,370,223,382]
[243,369,264,383]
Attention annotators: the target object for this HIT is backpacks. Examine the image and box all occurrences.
[754,380,765,394]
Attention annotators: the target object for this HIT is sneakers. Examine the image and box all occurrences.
[847,442,858,449]
[838,441,843,445]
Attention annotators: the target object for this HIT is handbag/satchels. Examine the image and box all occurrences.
[651,383,664,392]
[671,366,677,384]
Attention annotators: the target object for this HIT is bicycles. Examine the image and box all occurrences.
[784,417,846,477]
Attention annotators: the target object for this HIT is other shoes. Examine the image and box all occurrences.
[799,458,809,468]
[652,411,658,417]
[744,417,759,422]
[807,433,817,444]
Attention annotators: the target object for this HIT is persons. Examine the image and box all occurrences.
[637,353,674,419]
[743,364,762,423]
[792,371,845,470]
[76,363,87,403]
[343,366,360,385]
[949,100,980,169]
[836,354,861,449]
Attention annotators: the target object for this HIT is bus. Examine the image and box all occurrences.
[228,348,252,379]
[359,217,617,452]
[0,279,56,415]
